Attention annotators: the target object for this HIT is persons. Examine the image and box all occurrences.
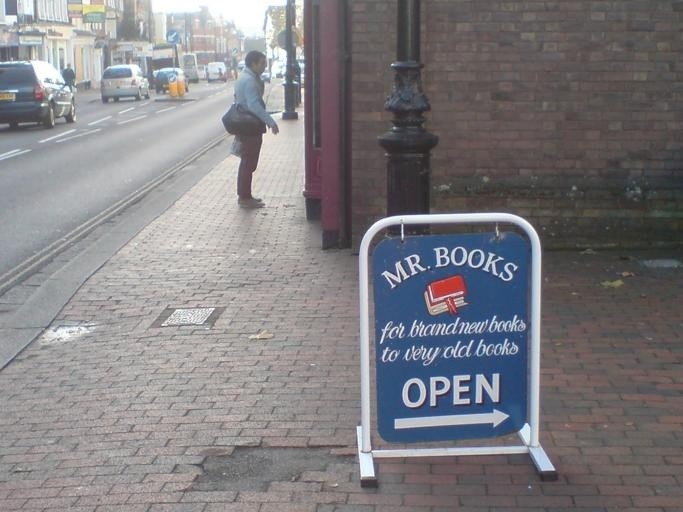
[233,50,279,209]
[61,63,75,92]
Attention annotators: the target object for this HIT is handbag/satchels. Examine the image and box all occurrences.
[228,136,244,157]
[221,102,267,134]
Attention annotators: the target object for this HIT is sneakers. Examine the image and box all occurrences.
[237,196,266,209]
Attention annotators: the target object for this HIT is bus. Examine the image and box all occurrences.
[183,53,201,84]
[150,43,186,91]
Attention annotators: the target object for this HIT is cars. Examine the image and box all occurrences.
[156,68,191,96]
[198,59,246,85]
[261,60,303,85]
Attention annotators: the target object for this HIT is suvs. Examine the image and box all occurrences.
[0,60,79,133]
[100,63,152,104]
